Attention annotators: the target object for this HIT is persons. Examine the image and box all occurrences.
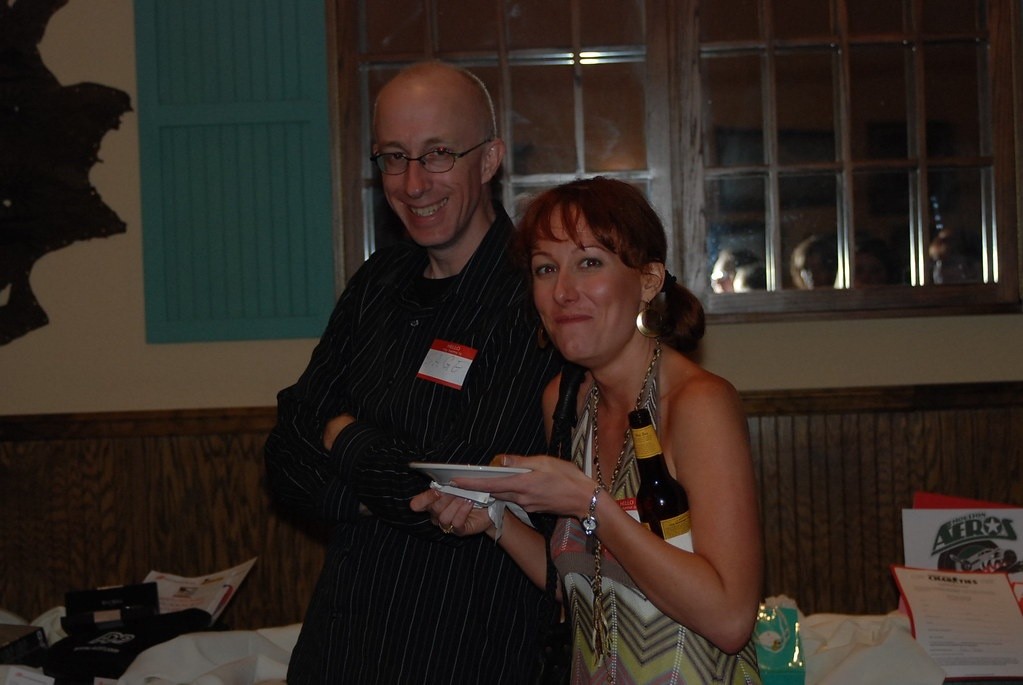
[265,60,572,685]
[409,175,765,685]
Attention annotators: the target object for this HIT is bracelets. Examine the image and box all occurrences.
[583,485,605,536]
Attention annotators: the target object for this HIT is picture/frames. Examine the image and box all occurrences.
[866,119,960,218]
[712,125,838,210]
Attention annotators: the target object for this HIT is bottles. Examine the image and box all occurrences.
[629,408,694,553]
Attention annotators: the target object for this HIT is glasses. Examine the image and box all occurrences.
[369,137,492,175]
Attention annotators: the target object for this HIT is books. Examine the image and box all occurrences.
[142,558,256,628]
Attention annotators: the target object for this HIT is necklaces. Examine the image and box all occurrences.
[593,337,662,660]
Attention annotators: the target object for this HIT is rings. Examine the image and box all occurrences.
[439,522,453,534]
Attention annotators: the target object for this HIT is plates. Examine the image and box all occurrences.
[409,462,533,487]
